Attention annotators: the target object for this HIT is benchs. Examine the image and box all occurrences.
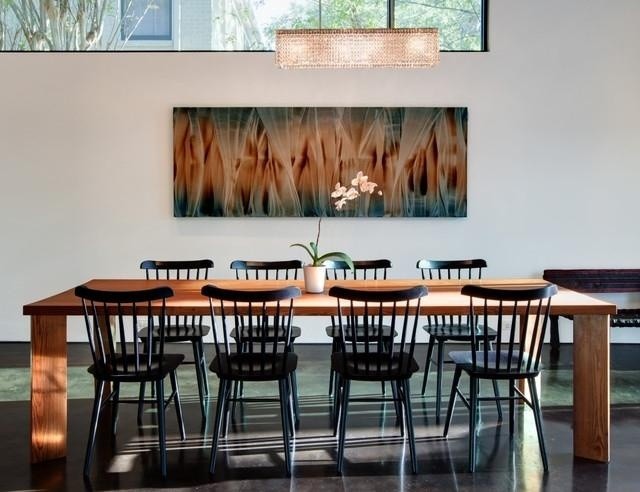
[541,267,640,365]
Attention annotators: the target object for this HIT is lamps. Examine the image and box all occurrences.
[272,0,442,74]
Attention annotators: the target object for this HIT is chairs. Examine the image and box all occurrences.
[73,283,186,477]
[133,257,213,431]
[416,257,504,424]
[446,282,559,481]
[324,258,406,437]
[199,283,302,478]
[229,260,304,425]
[326,283,431,475]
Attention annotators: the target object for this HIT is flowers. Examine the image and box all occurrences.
[289,168,385,274]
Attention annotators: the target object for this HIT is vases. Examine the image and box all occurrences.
[301,264,327,293]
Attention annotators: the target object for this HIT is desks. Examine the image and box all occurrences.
[24,272,617,466]
